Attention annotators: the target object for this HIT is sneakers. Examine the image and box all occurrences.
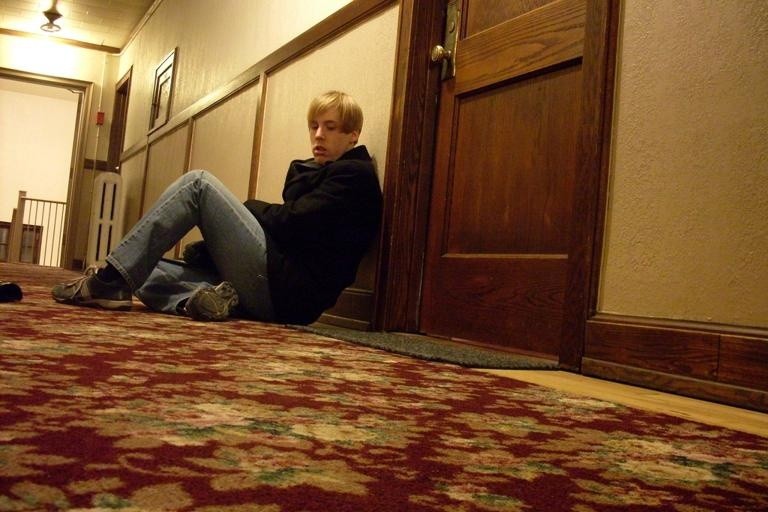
[51,263,132,312]
[185,281,240,322]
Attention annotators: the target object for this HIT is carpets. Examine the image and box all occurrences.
[284,319,562,370]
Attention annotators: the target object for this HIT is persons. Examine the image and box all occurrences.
[51,90,384,327]
